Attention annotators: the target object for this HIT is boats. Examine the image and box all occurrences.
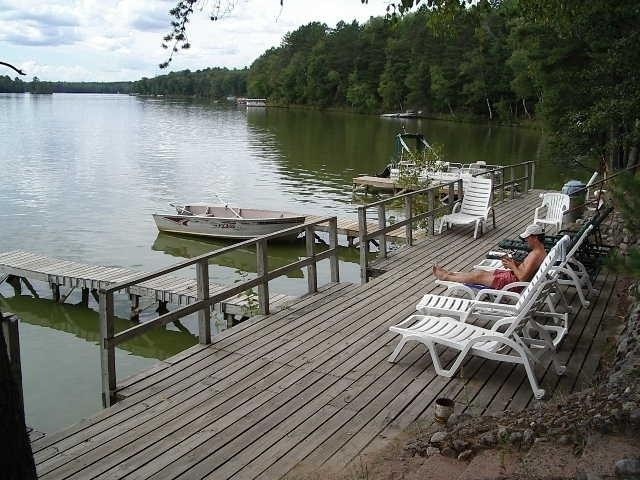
[154,200,306,243]
[380,137,504,178]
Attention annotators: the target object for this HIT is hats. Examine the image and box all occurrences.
[519,223,546,239]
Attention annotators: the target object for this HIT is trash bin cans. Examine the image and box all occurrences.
[562,180,586,223]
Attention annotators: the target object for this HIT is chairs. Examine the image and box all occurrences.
[586,172,598,201]
[533,192,570,233]
[438,177,495,238]
[441,163,503,192]
[389,224,599,398]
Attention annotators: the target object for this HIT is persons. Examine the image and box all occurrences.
[433,225,546,292]
